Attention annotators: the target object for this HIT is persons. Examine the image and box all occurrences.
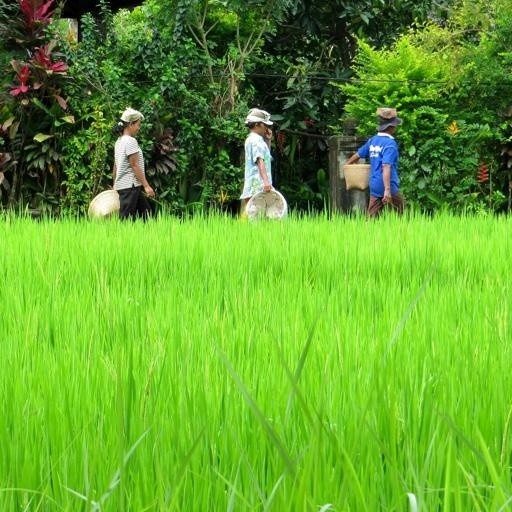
[240,108,274,216]
[345,117,405,220]
[112,109,155,223]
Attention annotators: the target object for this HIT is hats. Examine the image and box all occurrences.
[245,108,274,126]
[377,116,401,131]
[120,109,144,124]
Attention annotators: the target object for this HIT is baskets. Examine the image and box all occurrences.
[244,187,289,222]
[343,162,372,192]
[88,190,121,223]
